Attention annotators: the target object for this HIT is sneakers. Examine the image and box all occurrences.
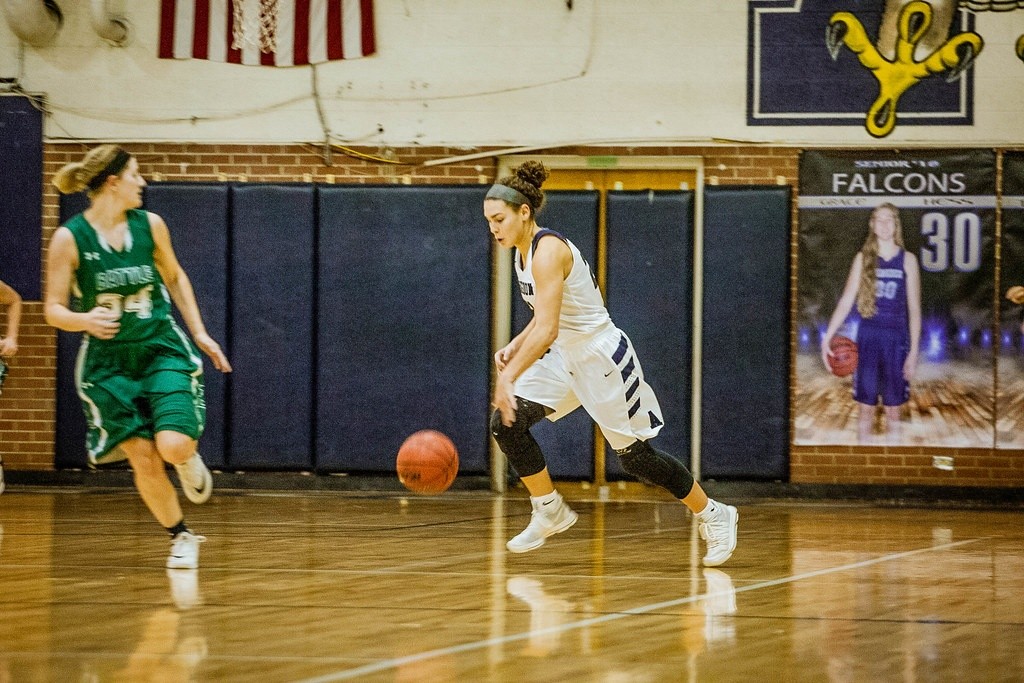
[698,499,739,566]
[173,451,214,505]
[165,529,208,569]
[505,495,578,553]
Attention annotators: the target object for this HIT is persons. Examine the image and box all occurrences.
[821,202,922,445]
[41,143,233,571]
[0,278,23,495]
[483,162,740,567]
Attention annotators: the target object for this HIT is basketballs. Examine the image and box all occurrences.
[827,337,859,377]
[396,428,459,494]
